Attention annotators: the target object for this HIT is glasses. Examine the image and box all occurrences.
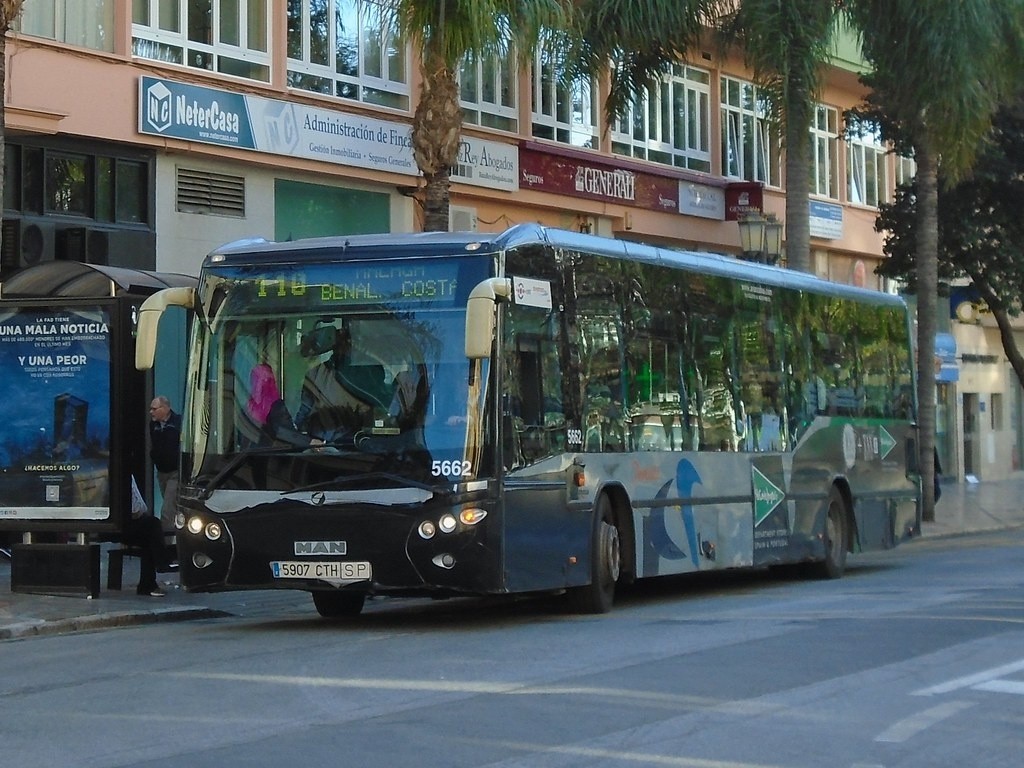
[151,408,159,410]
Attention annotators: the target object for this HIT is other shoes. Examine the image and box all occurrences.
[169,556,179,568]
[0,546,11,561]
[150,586,165,597]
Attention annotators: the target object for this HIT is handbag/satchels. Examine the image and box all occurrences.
[132,475,147,521]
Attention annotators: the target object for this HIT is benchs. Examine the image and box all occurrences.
[67,534,178,593]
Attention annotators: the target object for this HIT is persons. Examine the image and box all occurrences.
[417,364,483,426]
[149,395,183,544]
[98,506,179,597]
[236,363,324,453]
[296,327,372,446]
[933,445,943,505]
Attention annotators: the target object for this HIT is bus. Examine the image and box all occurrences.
[131,222,927,620]
